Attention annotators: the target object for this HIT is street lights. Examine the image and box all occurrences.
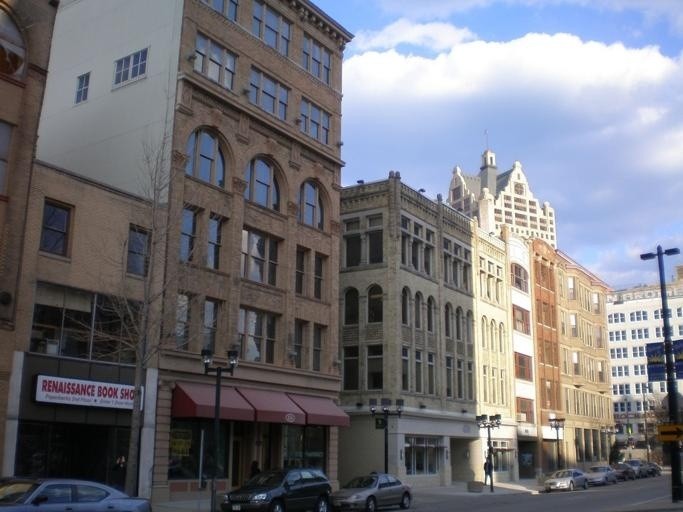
[199,342,243,512]
[599,423,619,466]
[474,412,501,492]
[368,404,404,474]
[637,242,679,505]
[546,416,565,471]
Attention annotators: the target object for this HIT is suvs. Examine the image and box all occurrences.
[216,465,336,512]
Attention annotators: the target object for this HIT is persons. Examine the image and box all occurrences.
[251,461,259,478]
[484,457,493,486]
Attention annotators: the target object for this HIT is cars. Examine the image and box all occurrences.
[584,465,617,486]
[0,475,154,512]
[326,471,413,512]
[542,468,588,492]
[611,458,664,481]
